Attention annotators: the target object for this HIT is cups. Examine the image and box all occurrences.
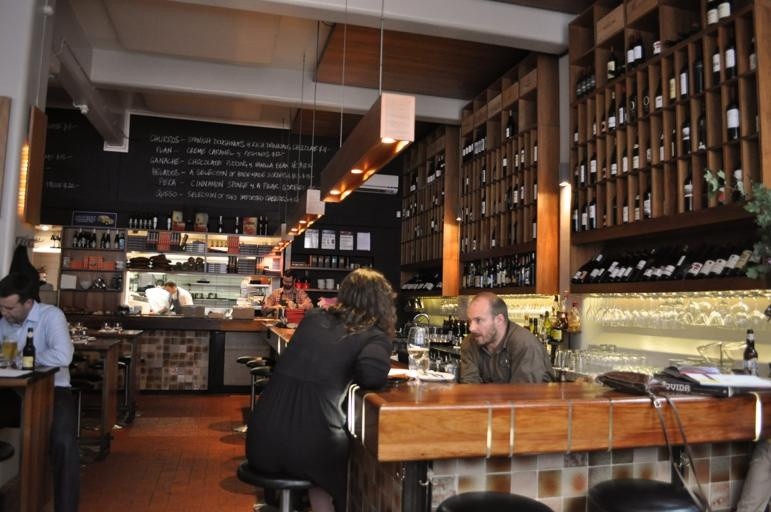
[102,322,112,331]
[584,292,767,329]
[2,340,17,370]
[724,342,746,362]
[552,348,648,377]
[697,342,722,365]
[115,322,123,332]
[67,321,87,341]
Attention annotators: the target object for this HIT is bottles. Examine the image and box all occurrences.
[227,256,237,273]
[568,240,764,285]
[442,313,469,347]
[115,230,119,249]
[116,273,123,289]
[462,252,534,288]
[219,216,223,233]
[234,216,239,234]
[572,0,758,229]
[55,231,60,248]
[294,270,302,288]
[111,274,117,288]
[167,217,171,230]
[400,272,442,290]
[91,227,97,248]
[743,329,758,376]
[402,154,444,238]
[146,231,158,251]
[173,257,203,272]
[258,215,263,235]
[119,233,125,249]
[170,233,188,251]
[263,215,268,235]
[459,107,537,250]
[51,232,54,248]
[72,228,91,248]
[22,328,36,371]
[101,232,107,248]
[302,270,311,289]
[106,229,110,248]
[521,294,581,344]
[128,217,157,230]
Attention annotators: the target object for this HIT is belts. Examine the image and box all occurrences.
[407,326,430,387]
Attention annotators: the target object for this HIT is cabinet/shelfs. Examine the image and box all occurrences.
[568,240,764,285]
[91,227,97,248]
[119,233,125,249]
[173,257,203,272]
[55,231,60,248]
[219,216,223,233]
[51,232,54,248]
[521,294,581,344]
[442,313,469,347]
[400,272,442,290]
[170,233,188,251]
[234,216,239,234]
[462,252,534,288]
[101,232,107,248]
[402,154,444,238]
[294,270,302,288]
[128,217,157,230]
[72,228,91,248]
[22,328,36,371]
[459,107,537,250]
[302,270,311,289]
[258,215,263,235]
[227,256,237,273]
[743,329,758,376]
[111,274,117,288]
[167,217,171,230]
[263,215,268,235]
[106,229,110,248]
[146,231,158,251]
[115,230,119,249]
[116,273,123,289]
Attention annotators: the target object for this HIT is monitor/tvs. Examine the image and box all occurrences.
[654,373,735,398]
[661,366,771,390]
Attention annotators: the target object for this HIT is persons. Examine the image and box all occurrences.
[145,280,174,313]
[262,270,313,315]
[737,440,771,512]
[246,268,397,512]
[0,273,80,512]
[164,281,193,312]
[459,292,554,384]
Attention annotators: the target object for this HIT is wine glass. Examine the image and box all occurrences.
[407,326,430,387]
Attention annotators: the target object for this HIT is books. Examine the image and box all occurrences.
[234,307,254,319]
[181,305,205,317]
[60,274,76,289]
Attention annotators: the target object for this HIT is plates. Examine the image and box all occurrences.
[569,0,771,292]
[122,229,285,317]
[400,127,458,296]
[420,371,456,381]
[459,51,558,295]
[57,226,126,316]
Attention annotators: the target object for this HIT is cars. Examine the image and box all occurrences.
[102,322,112,331]
[2,340,17,370]
[115,322,123,332]
[697,342,722,365]
[584,292,767,329]
[67,321,87,341]
[552,348,648,377]
[724,342,746,362]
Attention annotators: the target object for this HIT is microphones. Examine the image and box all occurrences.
[88,360,126,428]
[121,356,136,417]
[237,356,271,363]
[71,367,101,437]
[436,491,554,512]
[233,360,271,433]
[243,366,273,438]
[237,462,313,512]
[588,479,706,512]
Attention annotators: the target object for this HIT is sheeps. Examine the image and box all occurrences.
[414,313,431,327]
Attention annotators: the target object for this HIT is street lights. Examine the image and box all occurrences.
[598,371,716,512]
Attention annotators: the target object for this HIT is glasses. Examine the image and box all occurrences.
[319,0,415,204]
[272,199,294,253]
[287,55,324,235]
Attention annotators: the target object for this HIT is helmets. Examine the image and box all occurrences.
[420,371,456,381]
